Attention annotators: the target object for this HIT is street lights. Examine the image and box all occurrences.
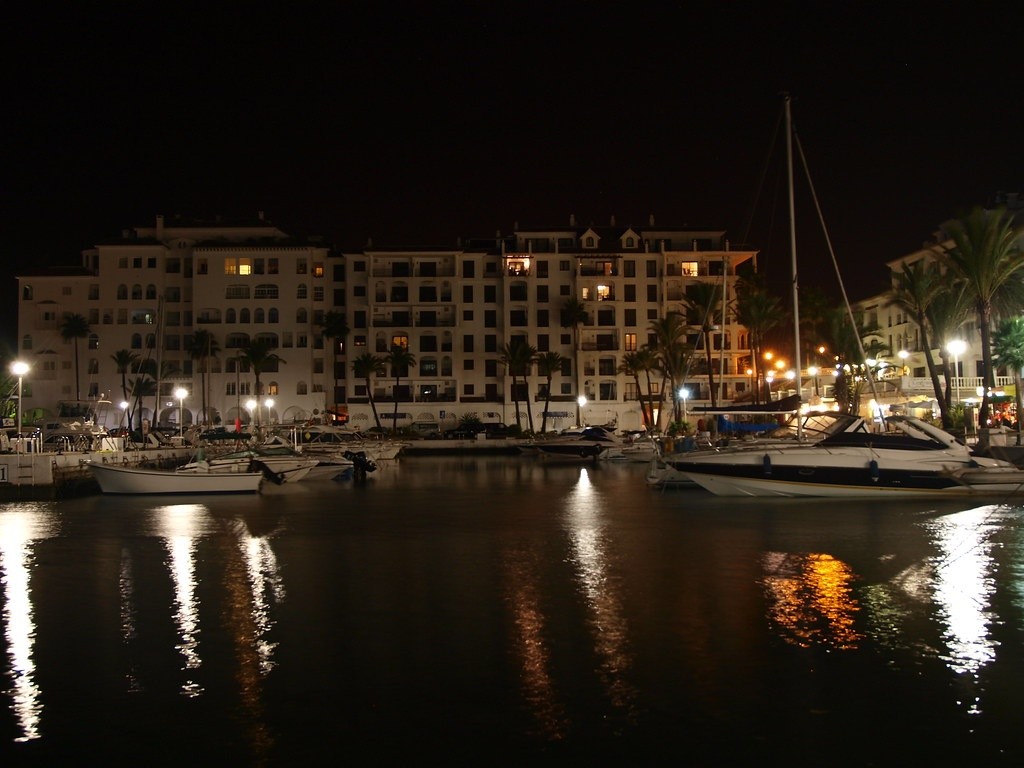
[172,387,189,446]
[679,388,690,424]
[944,336,971,406]
[10,360,32,436]
[263,398,275,426]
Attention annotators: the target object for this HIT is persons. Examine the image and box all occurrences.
[988,406,1018,429]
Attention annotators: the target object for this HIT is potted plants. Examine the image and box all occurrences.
[698,419,711,440]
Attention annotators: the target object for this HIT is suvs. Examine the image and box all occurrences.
[40,432,92,450]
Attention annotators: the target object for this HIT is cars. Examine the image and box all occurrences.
[97,428,122,438]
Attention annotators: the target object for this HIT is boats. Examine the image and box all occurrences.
[78,297,411,493]
[517,93,1024,497]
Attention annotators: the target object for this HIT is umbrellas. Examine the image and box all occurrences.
[901,399,940,416]
